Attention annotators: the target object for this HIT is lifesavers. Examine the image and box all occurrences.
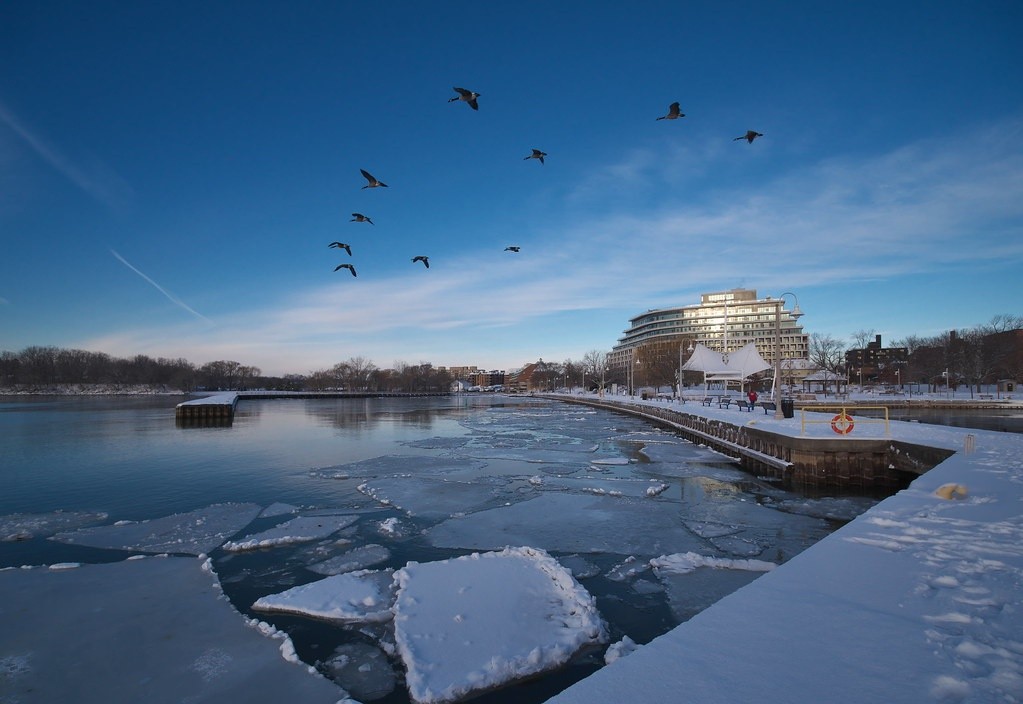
[832,414,854,434]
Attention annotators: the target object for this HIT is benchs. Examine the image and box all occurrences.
[760,401,777,415]
[719,399,732,409]
[735,399,754,412]
[703,398,713,406]
[678,397,685,404]
[666,396,675,403]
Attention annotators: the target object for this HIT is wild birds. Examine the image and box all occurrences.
[359,168,388,190]
[333,263,356,278]
[448,86,481,111]
[327,242,352,257]
[656,101,685,122]
[349,213,374,226]
[410,255,430,268]
[734,130,764,144]
[503,246,521,253]
[523,148,547,165]
